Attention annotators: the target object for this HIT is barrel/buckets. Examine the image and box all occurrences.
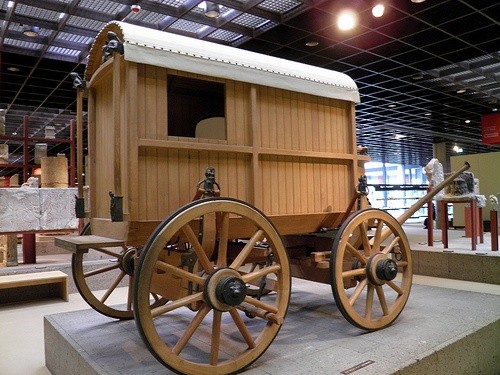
[41,157,68,188]
